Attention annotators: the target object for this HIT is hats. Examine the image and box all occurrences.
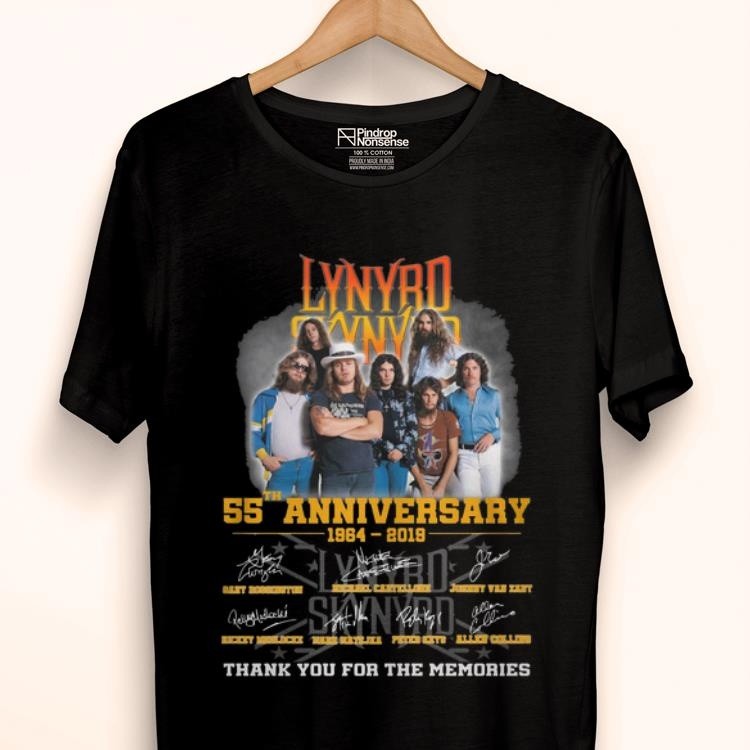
[320,342,364,370]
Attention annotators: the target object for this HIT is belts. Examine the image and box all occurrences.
[459,438,496,449]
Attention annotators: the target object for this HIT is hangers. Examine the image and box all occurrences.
[249,1,486,93]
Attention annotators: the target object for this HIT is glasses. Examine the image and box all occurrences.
[290,361,311,373]
